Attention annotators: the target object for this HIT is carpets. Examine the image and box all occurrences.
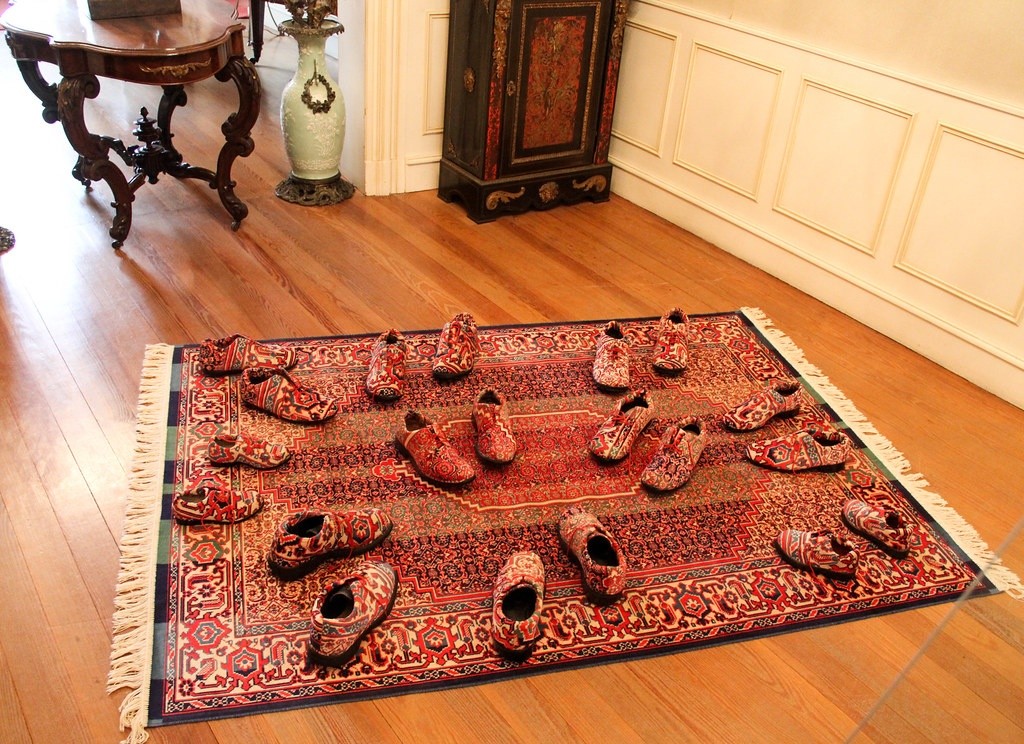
[98,301,1022,744]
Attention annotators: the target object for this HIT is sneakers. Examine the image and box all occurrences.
[492,551,546,660]
[267,508,394,579]
[395,409,476,487]
[472,386,516,464]
[199,332,299,375]
[653,307,690,373]
[305,562,398,666]
[640,415,707,492]
[745,428,850,473]
[721,380,801,433]
[556,506,627,603]
[209,435,291,469]
[775,529,860,581]
[589,390,652,462]
[241,366,337,423]
[841,499,913,559]
[171,486,264,524]
[432,312,477,376]
[366,328,407,399]
[592,321,630,391]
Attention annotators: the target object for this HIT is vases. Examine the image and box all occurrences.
[277,18,356,208]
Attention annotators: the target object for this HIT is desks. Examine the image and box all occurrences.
[1,0,264,252]
